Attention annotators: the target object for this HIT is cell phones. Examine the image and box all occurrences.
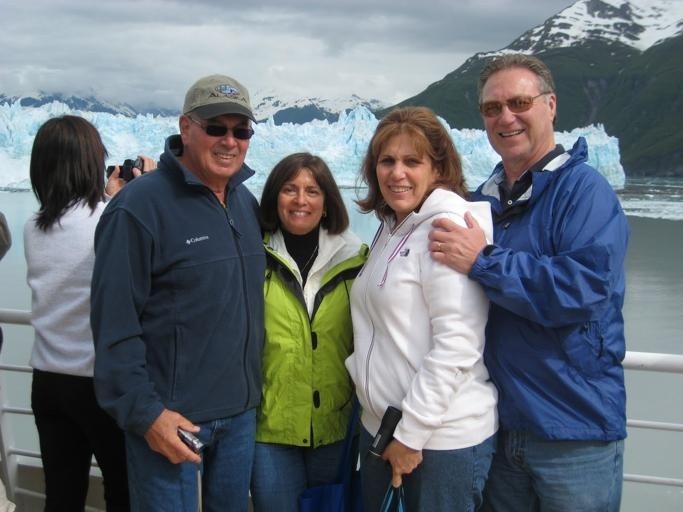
[177,426,208,454]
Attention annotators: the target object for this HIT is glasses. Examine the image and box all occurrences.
[183,112,255,141]
[475,89,554,119]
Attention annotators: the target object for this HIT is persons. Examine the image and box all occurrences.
[89,74,265,512]
[426,54,629,512]
[22,115,157,512]
[343,107,501,512]
[248,153,370,512]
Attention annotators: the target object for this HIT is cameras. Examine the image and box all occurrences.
[106,156,144,182]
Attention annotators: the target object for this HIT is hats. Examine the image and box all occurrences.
[181,72,259,127]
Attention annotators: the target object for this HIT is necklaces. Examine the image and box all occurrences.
[300,245,316,273]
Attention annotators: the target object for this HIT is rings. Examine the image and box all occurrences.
[436,242,443,251]
